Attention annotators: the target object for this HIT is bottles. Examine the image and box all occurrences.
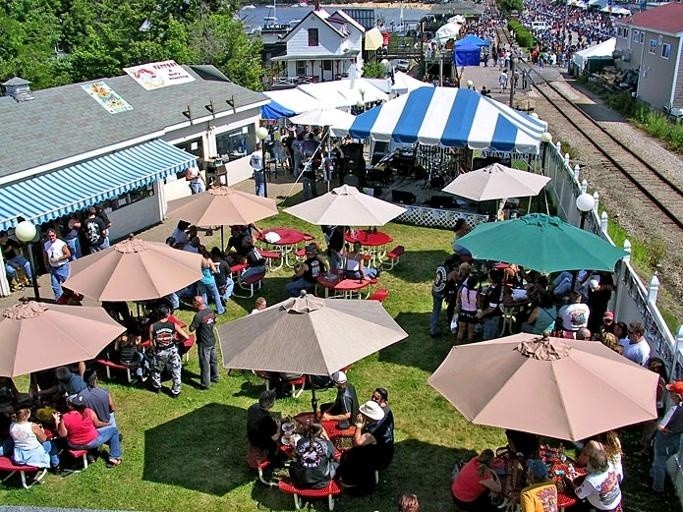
[567,463,576,481]
[556,440,568,467]
[49,250,55,265]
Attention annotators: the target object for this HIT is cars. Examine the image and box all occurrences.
[397,60,410,72]
[270,81,295,86]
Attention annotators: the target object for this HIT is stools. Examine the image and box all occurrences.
[247,446,380,511]
[245,227,404,305]
[95,313,195,385]
[487,494,624,512]
[0,445,112,489]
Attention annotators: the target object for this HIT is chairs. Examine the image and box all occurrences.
[3,248,30,291]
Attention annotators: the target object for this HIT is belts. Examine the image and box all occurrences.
[50,262,69,269]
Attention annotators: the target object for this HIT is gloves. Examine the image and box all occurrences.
[50,257,59,263]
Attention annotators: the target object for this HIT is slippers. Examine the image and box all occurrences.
[106,457,122,468]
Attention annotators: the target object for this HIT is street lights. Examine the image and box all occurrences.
[540,131,551,178]
[359,86,365,103]
[15,220,41,300]
[569,192,596,292]
[466,79,474,89]
[256,124,269,199]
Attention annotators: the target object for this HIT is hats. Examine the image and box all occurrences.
[358,400,385,421]
[666,379,683,396]
[525,458,546,478]
[602,311,614,321]
[333,371,348,384]
[377,387,388,401]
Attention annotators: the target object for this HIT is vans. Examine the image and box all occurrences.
[529,21,552,31]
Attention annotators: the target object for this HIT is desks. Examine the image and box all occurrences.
[293,411,356,442]
[480,285,531,336]
[227,263,249,301]
[479,474,530,512]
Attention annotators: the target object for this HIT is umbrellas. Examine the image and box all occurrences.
[288,107,356,127]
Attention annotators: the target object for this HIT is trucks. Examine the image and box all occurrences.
[510,9,519,18]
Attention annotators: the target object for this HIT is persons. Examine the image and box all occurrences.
[374,0,627,111]
[0,125,683,512]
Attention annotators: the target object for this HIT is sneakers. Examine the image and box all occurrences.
[630,438,663,496]
[28,280,41,288]
[55,464,64,475]
[430,332,441,339]
[171,390,180,399]
[151,385,162,394]
[194,383,207,390]
[210,378,219,383]
